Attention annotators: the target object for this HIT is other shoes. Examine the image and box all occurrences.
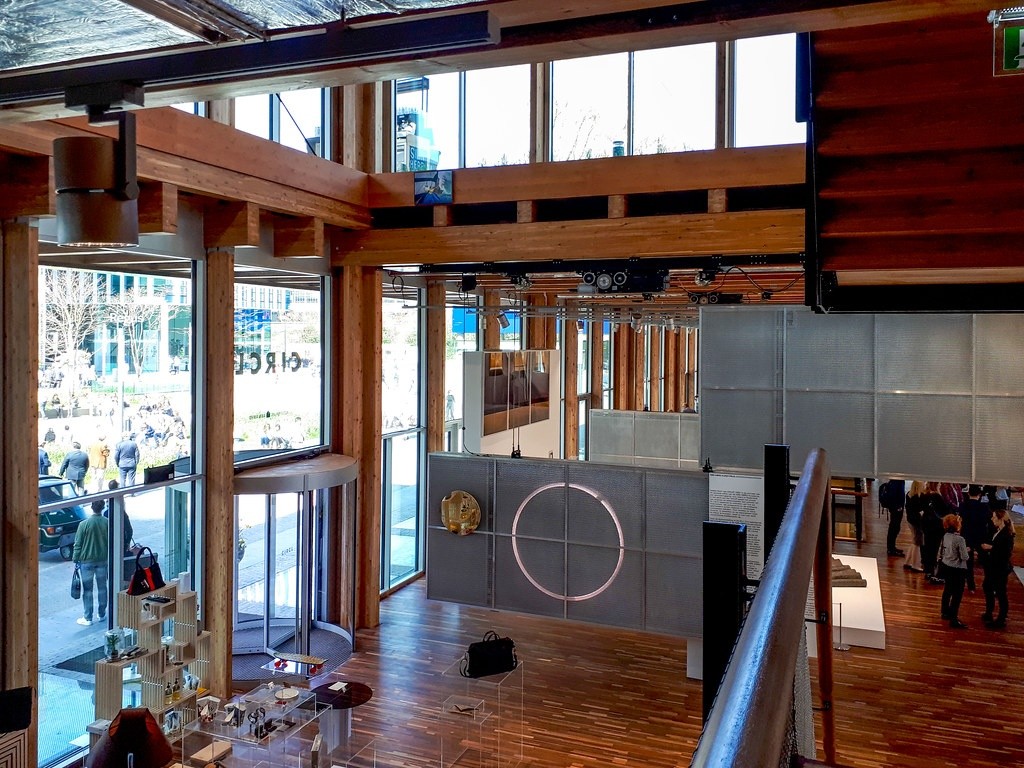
[902,564,923,573]
[942,613,951,620]
[887,548,906,558]
[948,621,968,628]
[926,574,944,584]
[981,612,993,619]
[984,618,1008,628]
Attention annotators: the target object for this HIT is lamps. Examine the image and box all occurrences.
[613,323,619,332]
[630,312,642,333]
[497,314,510,328]
[576,320,583,331]
[479,315,486,329]
[664,317,680,333]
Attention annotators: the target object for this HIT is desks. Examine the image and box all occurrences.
[311,682,373,764]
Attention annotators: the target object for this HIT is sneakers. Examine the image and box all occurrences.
[76,617,93,626]
[96,613,107,622]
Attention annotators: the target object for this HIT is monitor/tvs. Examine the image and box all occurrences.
[414,170,454,205]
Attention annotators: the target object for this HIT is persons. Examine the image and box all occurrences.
[446,390,455,421]
[938,514,970,628]
[903,475,1013,583]
[979,509,1016,629]
[71,498,111,626]
[103,498,133,559]
[878,479,908,557]
[36,364,188,498]
[258,420,294,452]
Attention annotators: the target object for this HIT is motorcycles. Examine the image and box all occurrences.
[38,475,87,561]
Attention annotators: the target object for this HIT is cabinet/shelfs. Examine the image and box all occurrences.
[86,581,211,748]
[184,684,331,768]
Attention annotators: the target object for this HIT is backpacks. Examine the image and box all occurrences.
[877,480,893,521]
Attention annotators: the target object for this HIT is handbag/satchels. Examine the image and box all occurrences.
[126,545,167,598]
[127,537,144,556]
[459,629,519,679]
[70,570,82,600]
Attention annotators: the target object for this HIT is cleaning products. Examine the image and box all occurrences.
[172,677,181,701]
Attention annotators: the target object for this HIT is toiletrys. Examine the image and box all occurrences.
[165,682,173,705]
